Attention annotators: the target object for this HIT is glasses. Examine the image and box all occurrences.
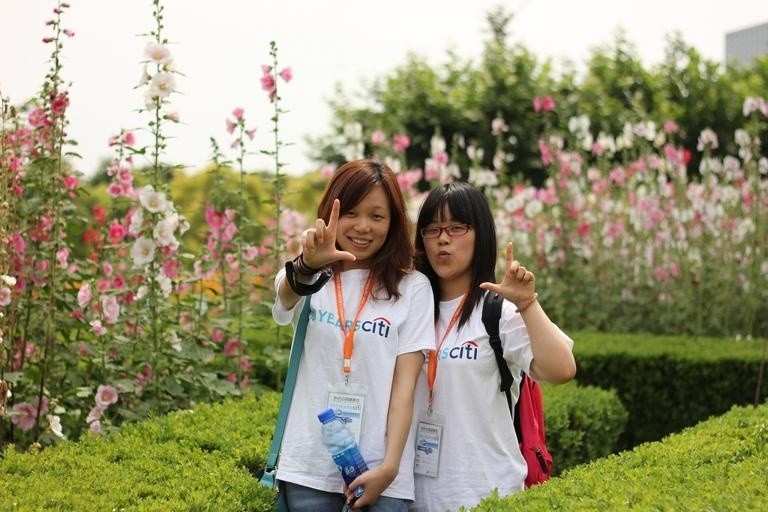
[420,224,473,237]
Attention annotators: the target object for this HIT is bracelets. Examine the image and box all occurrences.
[512,292,539,314]
[294,256,321,277]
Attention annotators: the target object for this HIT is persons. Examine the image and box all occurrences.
[406,178,582,512]
[255,156,438,512]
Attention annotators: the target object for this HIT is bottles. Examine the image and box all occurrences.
[318,409,369,497]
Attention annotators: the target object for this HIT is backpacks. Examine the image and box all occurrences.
[481,290,552,487]
